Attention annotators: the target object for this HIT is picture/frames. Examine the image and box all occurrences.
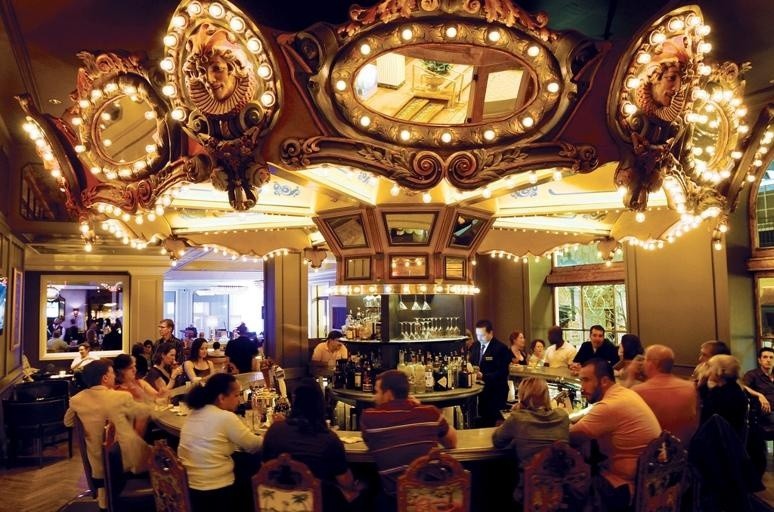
[10,265,23,352]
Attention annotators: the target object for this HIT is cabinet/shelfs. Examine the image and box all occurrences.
[411,59,473,109]
[328,294,485,431]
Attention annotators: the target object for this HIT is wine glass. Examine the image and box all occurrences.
[410,294,421,310]
[422,295,432,310]
[395,294,408,311]
[361,295,382,308]
[396,317,461,340]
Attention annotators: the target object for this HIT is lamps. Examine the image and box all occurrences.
[45,284,65,302]
[96,280,124,293]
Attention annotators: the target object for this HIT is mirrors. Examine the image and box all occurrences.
[441,254,469,284]
[373,202,447,252]
[388,251,429,280]
[340,255,376,283]
[40,274,129,360]
[314,205,377,261]
[437,203,495,256]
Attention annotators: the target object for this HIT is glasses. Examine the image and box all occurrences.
[158,325,166,329]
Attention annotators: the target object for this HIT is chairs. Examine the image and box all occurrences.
[523,439,591,512]
[397,447,471,512]
[251,453,321,512]
[742,397,750,448]
[207,353,227,363]
[691,414,732,512]
[2,398,73,458]
[102,416,155,512]
[634,429,689,512]
[742,385,774,440]
[15,380,70,409]
[74,411,105,499]
[147,438,191,512]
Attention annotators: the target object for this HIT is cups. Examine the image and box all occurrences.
[59,370,66,376]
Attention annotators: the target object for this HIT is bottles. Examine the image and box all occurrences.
[331,349,383,392]
[341,307,381,340]
[397,347,475,394]
[247,384,291,427]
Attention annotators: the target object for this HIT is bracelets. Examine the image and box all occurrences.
[170,377,176,381]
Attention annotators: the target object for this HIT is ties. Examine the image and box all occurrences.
[479,345,485,366]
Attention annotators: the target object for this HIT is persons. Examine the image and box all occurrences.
[225,323,259,373]
[143,340,154,368]
[112,354,168,404]
[177,338,215,383]
[102,319,112,335]
[102,325,122,352]
[136,356,166,395]
[81,330,102,351]
[88,319,103,338]
[65,319,80,342]
[569,359,671,511]
[141,343,187,392]
[85,314,94,329]
[622,345,705,475]
[155,319,185,384]
[737,347,774,491]
[193,49,238,104]
[527,339,547,372]
[467,320,512,414]
[505,332,530,368]
[610,334,645,382]
[114,317,122,334]
[48,331,68,355]
[182,330,195,350]
[690,340,745,398]
[698,356,755,452]
[70,343,95,373]
[208,342,225,358]
[45,318,65,342]
[63,363,160,508]
[645,39,694,114]
[491,376,570,507]
[359,370,458,512]
[261,379,370,512]
[129,341,148,378]
[177,372,283,510]
[543,326,578,374]
[568,324,620,375]
[311,331,349,377]
